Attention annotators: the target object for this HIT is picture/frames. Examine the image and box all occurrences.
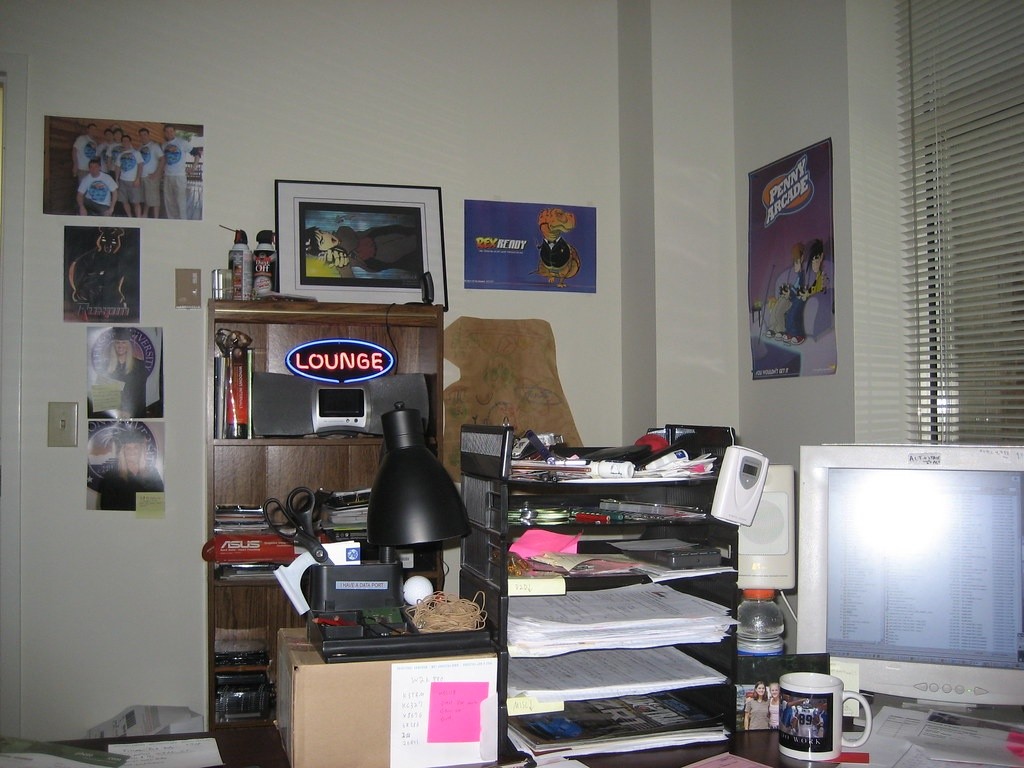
[275,179,448,311]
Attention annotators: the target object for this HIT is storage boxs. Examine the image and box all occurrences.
[276,626,498,767]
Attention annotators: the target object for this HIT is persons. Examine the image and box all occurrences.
[743,681,780,730]
[780,695,827,738]
[71,123,202,220]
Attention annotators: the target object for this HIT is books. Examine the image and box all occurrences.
[509,580,742,764]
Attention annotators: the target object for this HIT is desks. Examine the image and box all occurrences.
[48,723,1024,768]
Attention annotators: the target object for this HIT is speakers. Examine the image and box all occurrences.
[737,464,796,590]
[366,373,430,434]
[252,372,313,435]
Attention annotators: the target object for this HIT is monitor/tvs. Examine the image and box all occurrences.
[800,443,1024,712]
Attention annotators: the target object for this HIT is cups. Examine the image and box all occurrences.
[211,268,233,301]
[778,671,873,761]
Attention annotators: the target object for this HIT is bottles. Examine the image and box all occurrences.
[229,229,277,300]
[737,588,786,657]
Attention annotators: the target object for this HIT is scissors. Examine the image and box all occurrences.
[262,487,335,566]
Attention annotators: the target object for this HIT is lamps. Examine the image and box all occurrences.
[367,401,472,563]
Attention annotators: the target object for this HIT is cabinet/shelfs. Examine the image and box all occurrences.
[206,297,445,729]
[458,423,741,764]
